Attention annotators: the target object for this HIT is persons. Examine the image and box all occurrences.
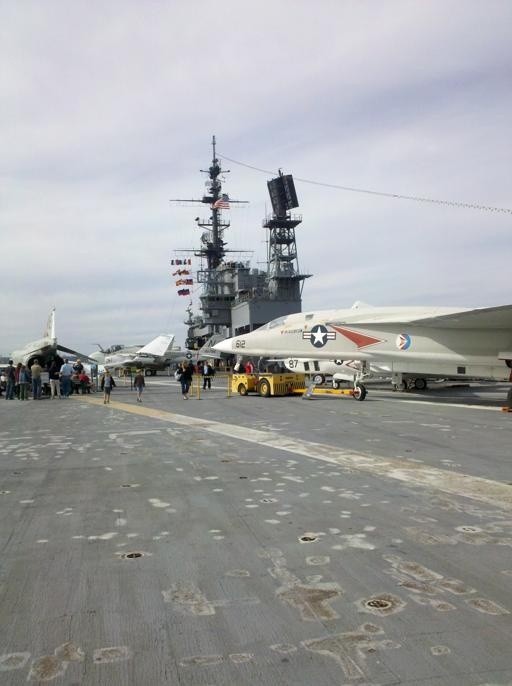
[188,360,197,375]
[4,356,90,400]
[100,370,116,404]
[176,361,193,401]
[134,369,146,403]
[201,361,214,391]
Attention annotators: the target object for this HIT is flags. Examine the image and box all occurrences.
[171,259,193,296]
[213,195,230,209]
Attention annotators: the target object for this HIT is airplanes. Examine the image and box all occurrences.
[0,305,99,386]
[209,301,512,400]
[264,356,393,390]
[89,333,222,376]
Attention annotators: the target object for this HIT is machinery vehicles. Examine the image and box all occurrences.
[230,360,306,398]
